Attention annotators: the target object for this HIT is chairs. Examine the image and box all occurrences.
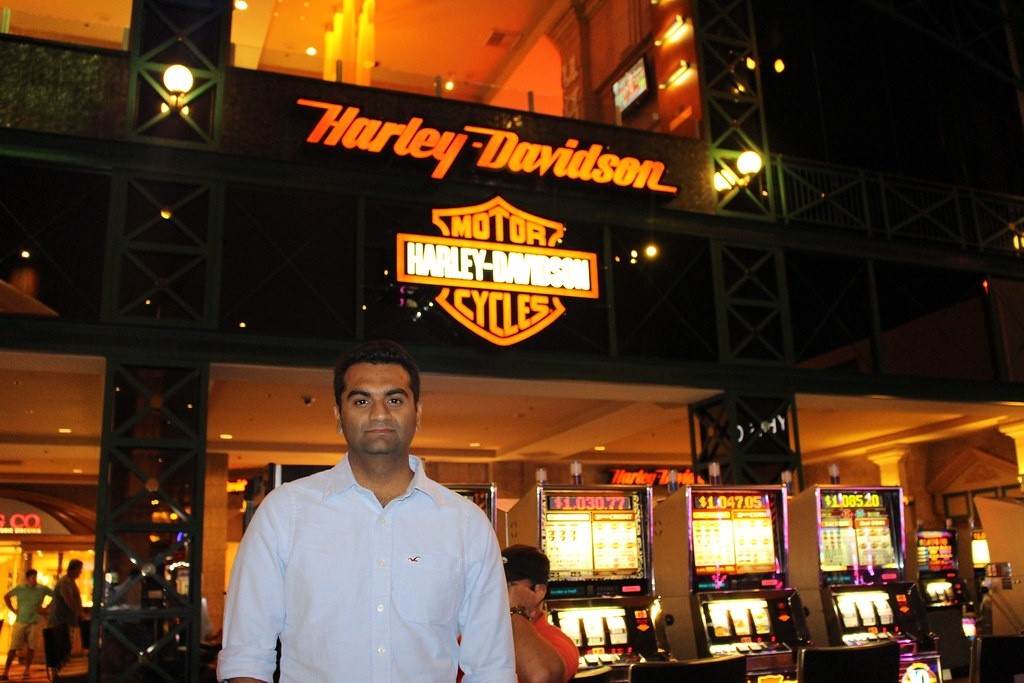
[969,634,1024,683]
[797,641,900,683]
[629,654,747,683]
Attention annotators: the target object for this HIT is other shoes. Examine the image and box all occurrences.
[0,675,9,681]
[22,673,30,678]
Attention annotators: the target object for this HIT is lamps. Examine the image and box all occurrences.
[659,59,688,89]
[654,15,683,46]
[163,64,193,116]
[737,148,762,186]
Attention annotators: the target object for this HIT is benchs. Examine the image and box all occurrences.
[42,621,89,683]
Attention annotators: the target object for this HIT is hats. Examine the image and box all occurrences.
[501,544,550,581]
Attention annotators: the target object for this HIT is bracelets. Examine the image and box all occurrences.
[510,604,534,622]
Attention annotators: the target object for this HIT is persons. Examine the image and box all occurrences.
[0,569,55,680]
[43,559,87,679]
[456,543,578,683]
[216,337,520,683]
